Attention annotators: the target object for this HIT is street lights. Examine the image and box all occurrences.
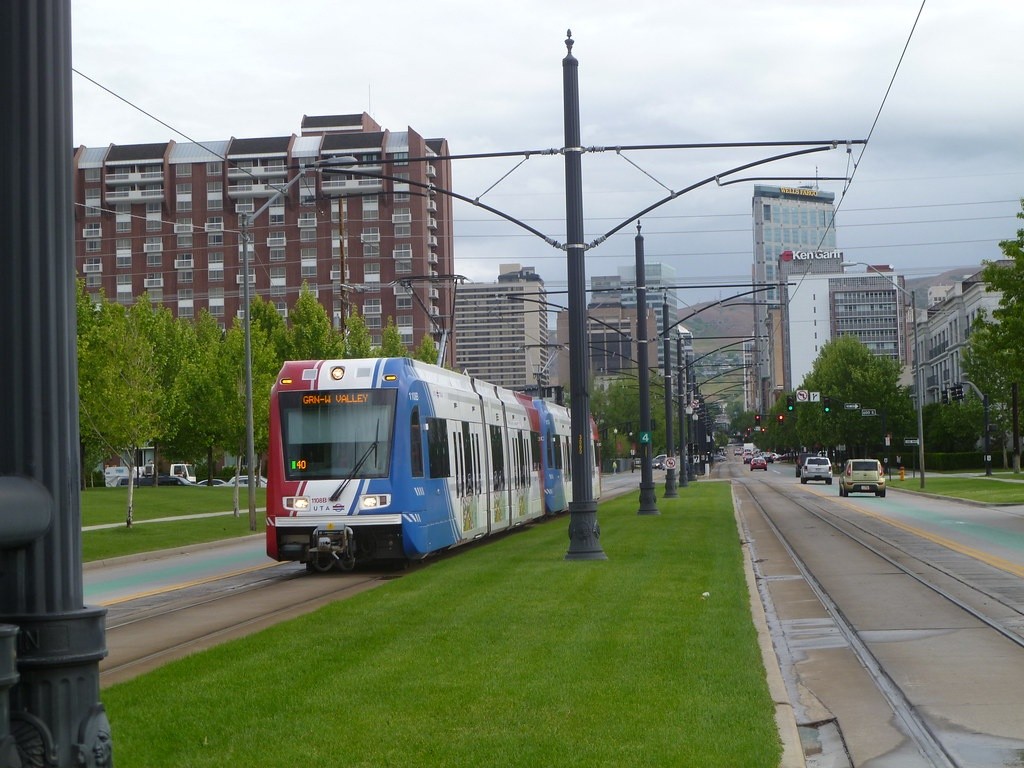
[244,155,358,535]
[839,261,925,488]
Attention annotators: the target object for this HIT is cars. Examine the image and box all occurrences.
[750,457,767,471]
[839,459,887,497]
[226,474,268,487]
[652,460,662,470]
[800,457,833,485]
[634,458,641,465]
[733,447,781,464]
[196,479,226,486]
[777,453,795,462]
[714,455,727,462]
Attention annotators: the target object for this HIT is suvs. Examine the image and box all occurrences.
[795,453,817,477]
[140,476,196,486]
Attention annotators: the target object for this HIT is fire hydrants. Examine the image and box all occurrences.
[898,466,905,481]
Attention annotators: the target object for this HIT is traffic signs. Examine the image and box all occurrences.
[844,403,862,410]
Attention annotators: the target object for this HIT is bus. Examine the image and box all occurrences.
[266,271,601,575]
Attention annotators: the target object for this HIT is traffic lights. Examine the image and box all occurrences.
[778,414,784,425]
[787,394,796,412]
[755,415,761,426]
[956,385,965,401]
[823,397,831,413]
[951,386,957,401]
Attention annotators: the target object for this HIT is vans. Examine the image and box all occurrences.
[817,450,828,458]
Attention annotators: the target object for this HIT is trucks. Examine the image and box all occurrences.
[119,463,196,486]
